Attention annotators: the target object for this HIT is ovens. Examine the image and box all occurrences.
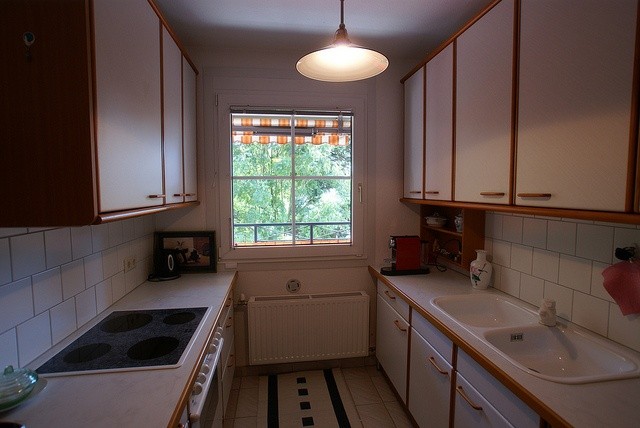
[185,326,239,427]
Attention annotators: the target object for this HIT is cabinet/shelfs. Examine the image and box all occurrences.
[452,346,542,427]
[62,2,164,227]
[218,299,237,413]
[374,279,407,409]
[163,22,200,210]
[456,0,637,215]
[408,308,454,428]
[401,40,454,207]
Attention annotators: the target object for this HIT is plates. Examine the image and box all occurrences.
[1,379,49,413]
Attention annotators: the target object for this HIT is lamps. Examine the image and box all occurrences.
[296,0,389,85]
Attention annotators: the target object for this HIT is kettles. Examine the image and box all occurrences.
[150,248,187,281]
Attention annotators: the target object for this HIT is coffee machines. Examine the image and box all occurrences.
[380,234,431,276]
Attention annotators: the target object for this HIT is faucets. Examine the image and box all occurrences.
[537,299,559,328]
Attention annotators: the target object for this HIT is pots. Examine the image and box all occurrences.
[422,211,448,230]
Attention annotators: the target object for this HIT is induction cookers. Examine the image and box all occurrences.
[34,304,213,377]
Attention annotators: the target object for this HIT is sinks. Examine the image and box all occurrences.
[435,294,542,328]
[483,323,640,383]
